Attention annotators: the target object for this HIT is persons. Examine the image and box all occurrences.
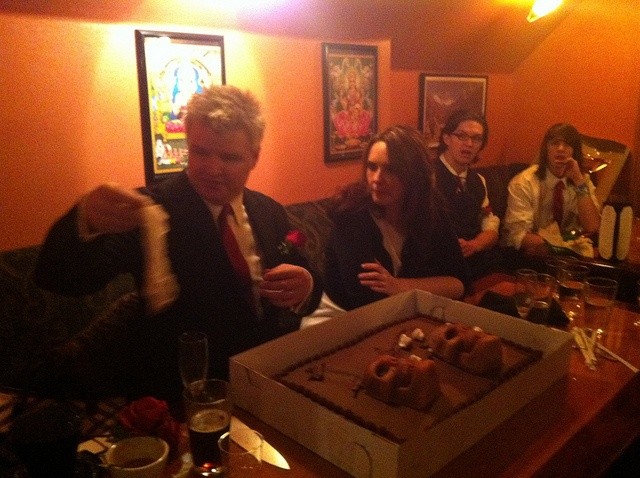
[505,127,601,257]
[328,125,474,303]
[32,83,323,371]
[426,109,500,265]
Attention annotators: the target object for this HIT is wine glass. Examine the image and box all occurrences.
[177,329,209,400]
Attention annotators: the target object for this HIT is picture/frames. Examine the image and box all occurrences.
[321,39,385,163]
[134,26,225,190]
[416,68,490,151]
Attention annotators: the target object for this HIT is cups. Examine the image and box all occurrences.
[582,276,618,341]
[218,429,264,478]
[530,274,556,310]
[183,379,233,478]
[515,268,537,313]
[559,265,590,321]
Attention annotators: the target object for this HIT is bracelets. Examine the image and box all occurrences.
[574,178,591,198]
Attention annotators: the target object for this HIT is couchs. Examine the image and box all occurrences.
[0,163,629,391]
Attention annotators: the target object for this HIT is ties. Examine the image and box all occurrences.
[552,181,565,227]
[218,202,251,285]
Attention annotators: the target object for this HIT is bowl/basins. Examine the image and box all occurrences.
[105,436,170,478]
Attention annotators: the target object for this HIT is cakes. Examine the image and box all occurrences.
[269,312,543,444]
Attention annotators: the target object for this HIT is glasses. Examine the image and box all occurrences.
[449,130,483,142]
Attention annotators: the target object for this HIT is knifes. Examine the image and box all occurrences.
[229,415,291,470]
